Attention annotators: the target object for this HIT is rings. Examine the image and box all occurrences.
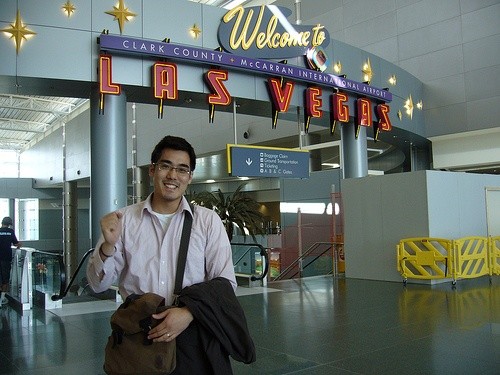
[167,333,170,338]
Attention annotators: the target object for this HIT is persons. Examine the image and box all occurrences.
[85,136,257,375]
[0,217,22,304]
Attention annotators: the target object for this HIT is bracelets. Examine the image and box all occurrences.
[101,242,117,257]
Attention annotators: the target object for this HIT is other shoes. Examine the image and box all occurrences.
[0,297,8,307]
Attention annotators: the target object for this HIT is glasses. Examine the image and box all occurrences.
[153,162,190,176]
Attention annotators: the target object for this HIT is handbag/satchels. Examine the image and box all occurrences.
[104,294,176,375]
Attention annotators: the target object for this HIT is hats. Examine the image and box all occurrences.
[2,217,12,225]
